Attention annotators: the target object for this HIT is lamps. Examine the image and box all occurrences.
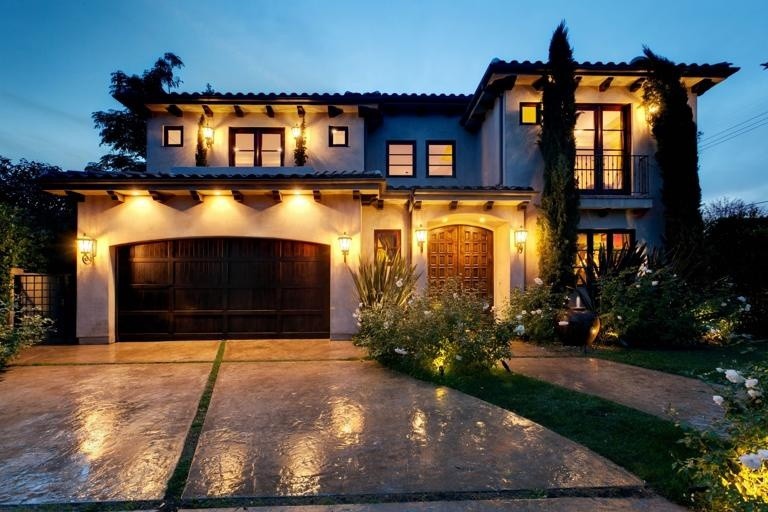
[415,223,427,254]
[338,231,351,263]
[201,120,214,148]
[291,123,301,141]
[76,233,96,266]
[514,224,528,255]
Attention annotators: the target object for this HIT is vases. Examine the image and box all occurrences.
[569,307,600,346]
